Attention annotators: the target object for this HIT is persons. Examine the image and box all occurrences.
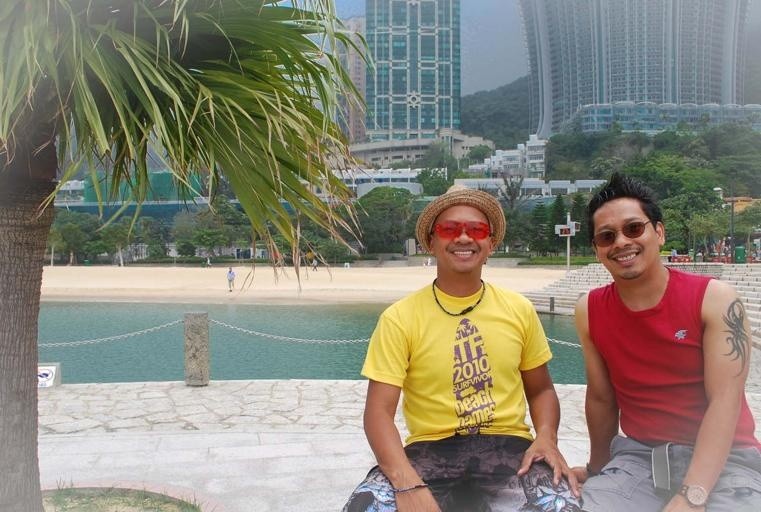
[226,267,236,292]
[207,257,212,269]
[311,259,318,272]
[696,250,702,257]
[568,171,761,512]
[341,182,586,511]
[667,247,677,262]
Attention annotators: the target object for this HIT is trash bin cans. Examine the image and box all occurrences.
[688,249,696,262]
[735,246,746,263]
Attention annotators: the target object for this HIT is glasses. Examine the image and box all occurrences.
[593,221,651,247]
[432,220,493,239]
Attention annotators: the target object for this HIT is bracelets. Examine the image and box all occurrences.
[392,483,428,492]
[586,462,593,477]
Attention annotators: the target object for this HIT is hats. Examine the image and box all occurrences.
[417,185,505,252]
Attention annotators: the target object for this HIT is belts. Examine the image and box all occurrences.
[652,443,669,492]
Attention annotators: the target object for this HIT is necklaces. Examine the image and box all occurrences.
[432,277,486,316]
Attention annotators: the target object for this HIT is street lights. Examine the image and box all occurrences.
[726,165,738,264]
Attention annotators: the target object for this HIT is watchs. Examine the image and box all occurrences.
[678,484,710,507]
